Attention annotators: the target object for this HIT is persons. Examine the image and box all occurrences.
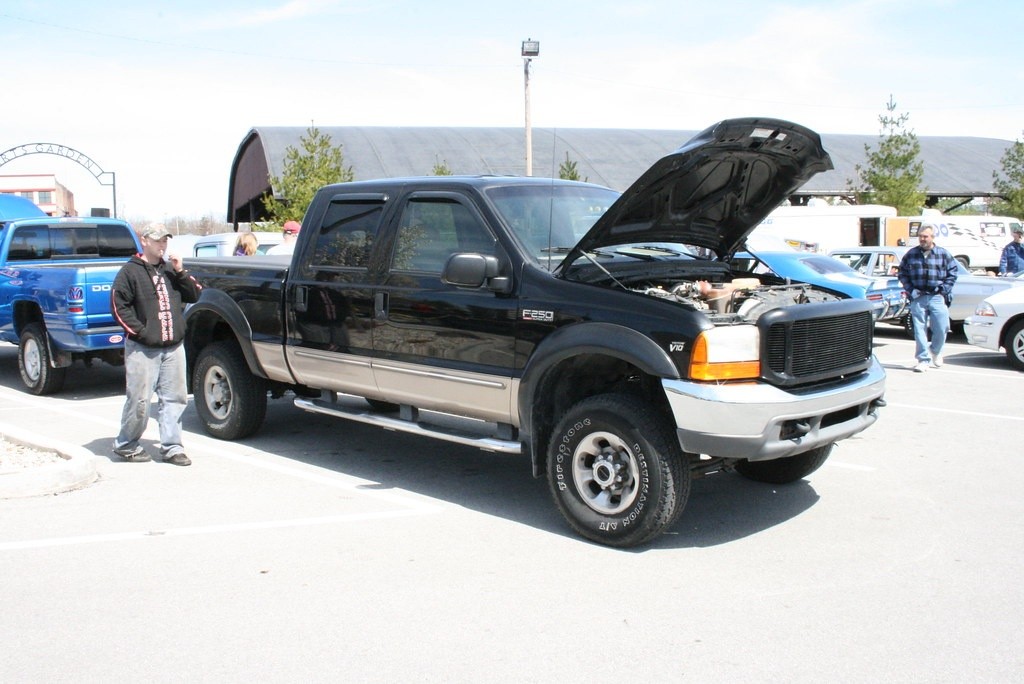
[232,232,259,257]
[266,220,302,255]
[897,225,958,371]
[110,224,202,467]
[998,229,1024,277]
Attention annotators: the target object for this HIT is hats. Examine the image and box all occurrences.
[142,224,174,240]
[284,222,300,234]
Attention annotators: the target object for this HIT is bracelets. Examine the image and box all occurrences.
[177,268,184,272]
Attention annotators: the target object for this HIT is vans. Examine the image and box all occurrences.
[749,190,1024,276]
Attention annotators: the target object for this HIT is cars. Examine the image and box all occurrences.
[709,251,909,327]
[188,231,296,260]
[962,272,1024,372]
[162,114,889,548]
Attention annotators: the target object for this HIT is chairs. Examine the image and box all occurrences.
[8,244,38,260]
[76,240,106,254]
[409,223,448,268]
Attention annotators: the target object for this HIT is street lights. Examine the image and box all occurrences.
[521,34,549,175]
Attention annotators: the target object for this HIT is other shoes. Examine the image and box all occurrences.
[162,454,191,466]
[114,446,152,462]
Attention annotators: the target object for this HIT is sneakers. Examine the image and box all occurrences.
[929,347,943,366]
[914,362,931,372]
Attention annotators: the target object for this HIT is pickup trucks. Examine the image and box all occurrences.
[0,195,144,397]
